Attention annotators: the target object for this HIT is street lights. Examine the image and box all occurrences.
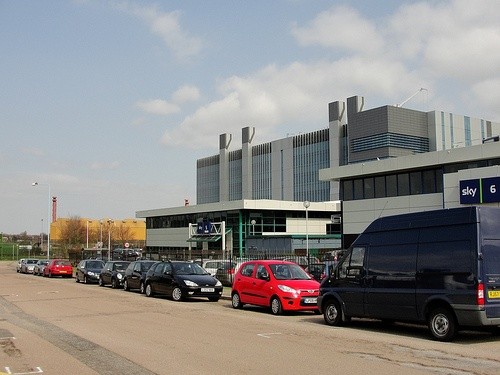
[251,220,256,236]
[121,219,126,242]
[32,182,51,259]
[304,200,310,258]
[100,220,102,248]
[126,218,136,242]
[107,219,114,260]
[86,220,92,248]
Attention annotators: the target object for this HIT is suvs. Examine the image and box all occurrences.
[304,261,345,283]
[324,249,349,261]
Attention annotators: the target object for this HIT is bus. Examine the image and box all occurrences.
[82,247,112,260]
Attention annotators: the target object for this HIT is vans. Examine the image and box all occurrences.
[314,204,500,342]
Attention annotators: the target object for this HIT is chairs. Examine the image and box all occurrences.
[246,268,253,277]
[277,266,285,279]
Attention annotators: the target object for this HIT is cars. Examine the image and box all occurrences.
[75,259,106,283]
[43,259,72,278]
[194,258,260,286]
[145,260,224,302]
[124,260,160,293]
[230,260,321,315]
[16,258,39,274]
[274,256,321,265]
[99,260,132,289]
[33,259,59,275]
